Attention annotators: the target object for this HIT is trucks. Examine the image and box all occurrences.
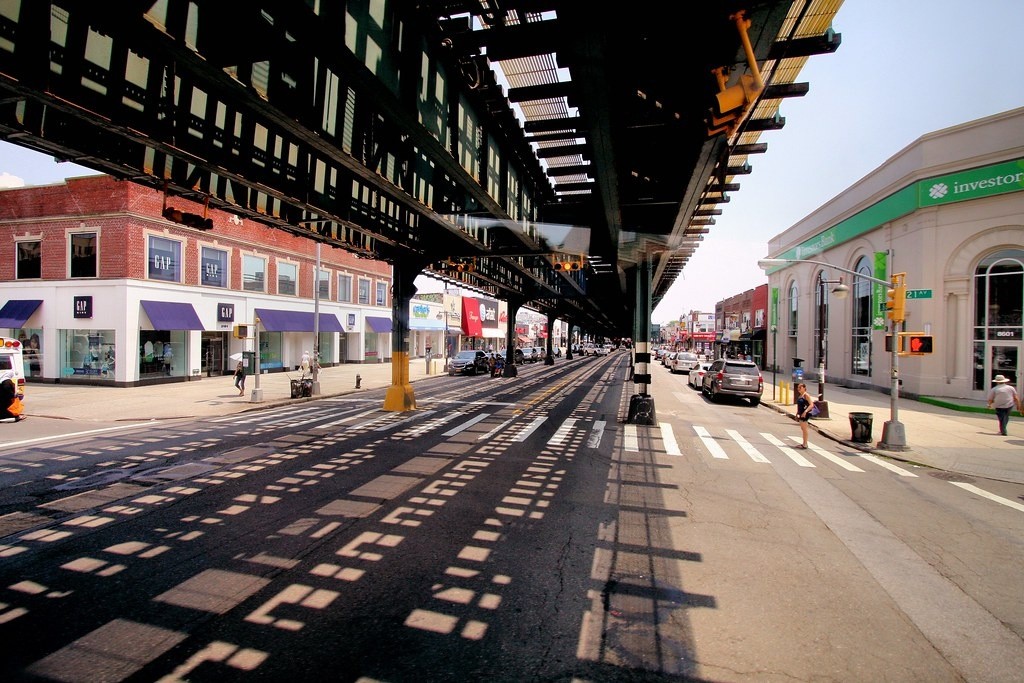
[0,336,26,418]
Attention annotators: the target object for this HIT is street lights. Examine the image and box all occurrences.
[757,257,908,450]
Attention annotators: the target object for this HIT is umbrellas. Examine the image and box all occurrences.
[229,352,242,362]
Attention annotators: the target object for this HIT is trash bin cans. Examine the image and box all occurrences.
[291,379,303,398]
[850,412,873,442]
[302,379,313,397]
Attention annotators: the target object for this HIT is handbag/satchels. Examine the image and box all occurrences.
[804,393,820,416]
[299,366,302,373]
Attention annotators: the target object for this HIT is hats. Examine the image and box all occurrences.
[992,375,1010,382]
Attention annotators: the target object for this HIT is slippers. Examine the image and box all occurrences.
[797,444,807,449]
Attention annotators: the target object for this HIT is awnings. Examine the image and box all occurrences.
[365,316,393,334]
[0,299,43,329]
[140,300,206,331]
[738,327,767,342]
[519,334,532,343]
[254,307,345,334]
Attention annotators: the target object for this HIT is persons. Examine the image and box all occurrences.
[704,347,711,362]
[83,345,116,378]
[233,361,246,396]
[17,329,42,377]
[477,343,505,353]
[145,340,174,376]
[300,351,321,378]
[0,370,27,423]
[795,383,814,449]
[488,353,497,378]
[987,375,1020,436]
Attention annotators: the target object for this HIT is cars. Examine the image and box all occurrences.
[443,341,765,411]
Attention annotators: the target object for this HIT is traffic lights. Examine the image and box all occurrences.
[883,331,934,356]
[233,325,249,340]
[886,273,906,325]
